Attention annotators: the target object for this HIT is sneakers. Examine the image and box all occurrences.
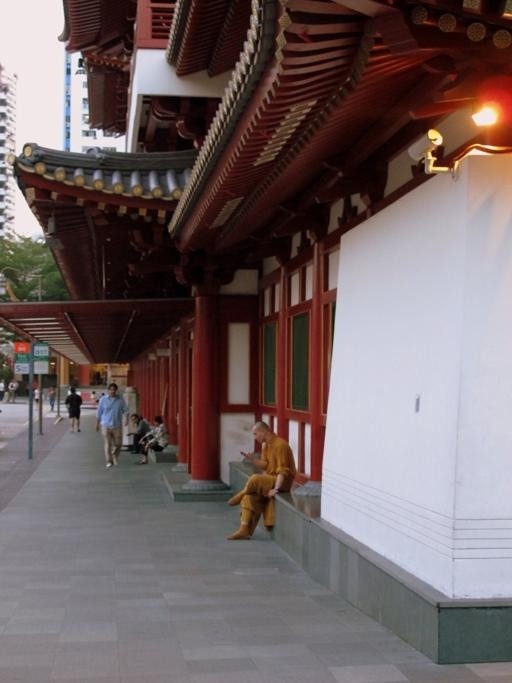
[226,524,251,540]
[228,491,243,506]
[105,457,148,469]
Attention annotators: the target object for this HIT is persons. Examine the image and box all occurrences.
[65,387,84,432]
[0,378,5,400]
[96,382,129,469]
[224,419,297,541]
[34,386,39,403]
[127,413,151,452]
[4,378,19,403]
[47,385,57,412]
[137,415,169,464]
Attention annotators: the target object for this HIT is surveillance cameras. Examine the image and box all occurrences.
[406,129,443,161]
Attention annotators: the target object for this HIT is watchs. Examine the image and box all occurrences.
[274,486,280,490]
